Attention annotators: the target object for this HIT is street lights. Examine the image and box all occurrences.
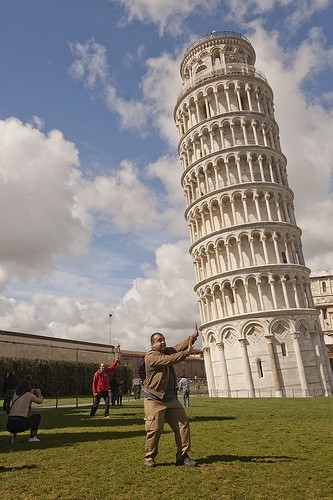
[108,313,113,345]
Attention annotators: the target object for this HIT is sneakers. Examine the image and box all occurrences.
[28,436,40,442]
[145,459,155,467]
[8,433,14,445]
[176,456,197,466]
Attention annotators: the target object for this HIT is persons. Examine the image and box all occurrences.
[6,369,18,414]
[2,373,11,412]
[6,378,43,444]
[88,355,120,419]
[23,374,34,413]
[110,375,118,406]
[132,383,138,399]
[142,322,199,468]
[136,383,142,400]
[177,374,190,408]
[116,376,123,406]
